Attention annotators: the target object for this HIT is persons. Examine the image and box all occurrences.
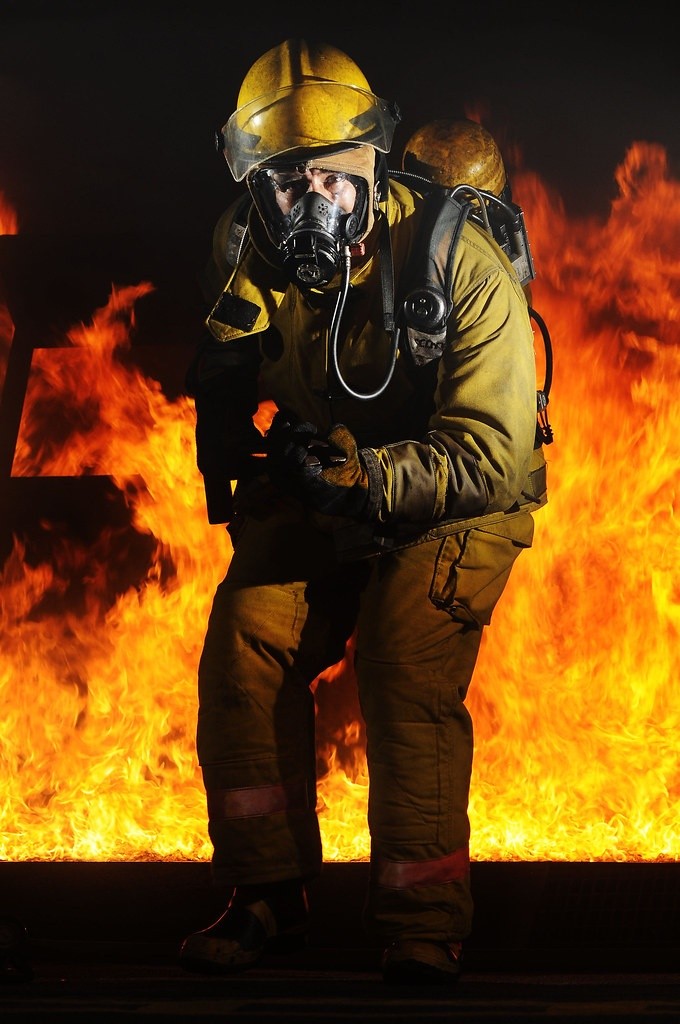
[168,40,538,967]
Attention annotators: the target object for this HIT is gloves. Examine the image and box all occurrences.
[264,408,368,520]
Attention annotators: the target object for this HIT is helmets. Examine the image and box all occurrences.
[212,33,395,165]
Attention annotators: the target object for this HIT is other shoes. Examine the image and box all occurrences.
[379,938,461,976]
[178,880,312,970]
